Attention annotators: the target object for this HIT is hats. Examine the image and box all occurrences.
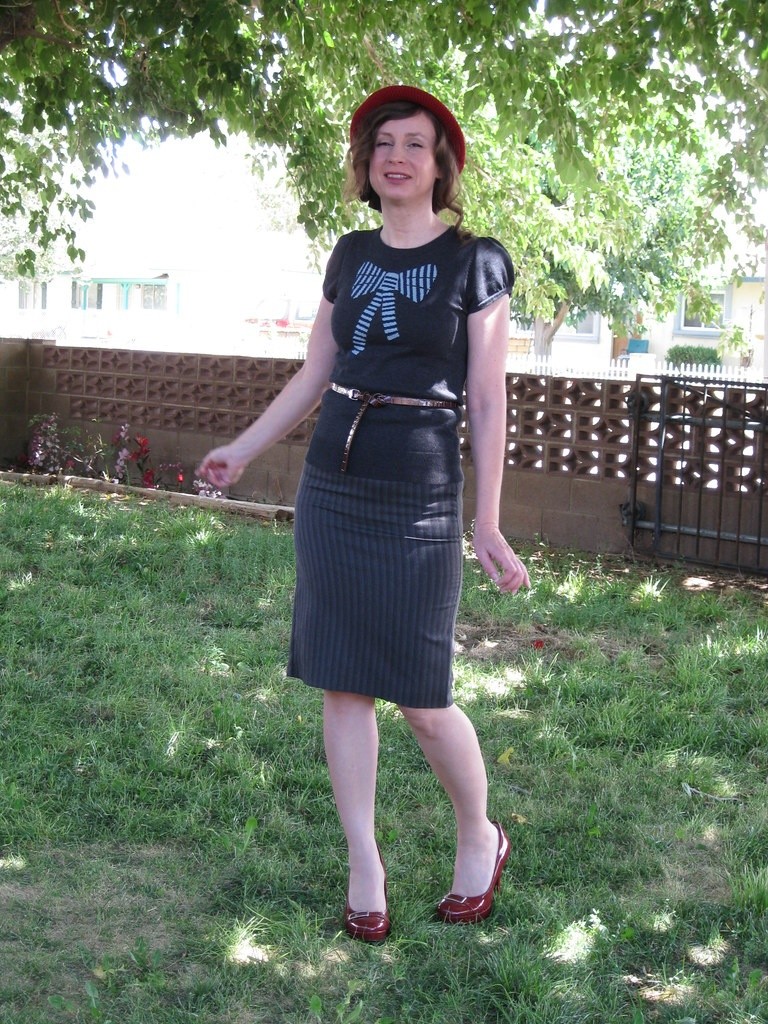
[349,84,466,173]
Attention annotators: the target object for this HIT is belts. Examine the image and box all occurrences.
[324,380,456,473]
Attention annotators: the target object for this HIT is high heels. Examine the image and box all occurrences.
[345,844,390,941]
[438,819,512,927]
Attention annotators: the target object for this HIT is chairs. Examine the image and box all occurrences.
[621,338,649,355]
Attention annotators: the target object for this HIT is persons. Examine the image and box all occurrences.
[197,86,532,942]
